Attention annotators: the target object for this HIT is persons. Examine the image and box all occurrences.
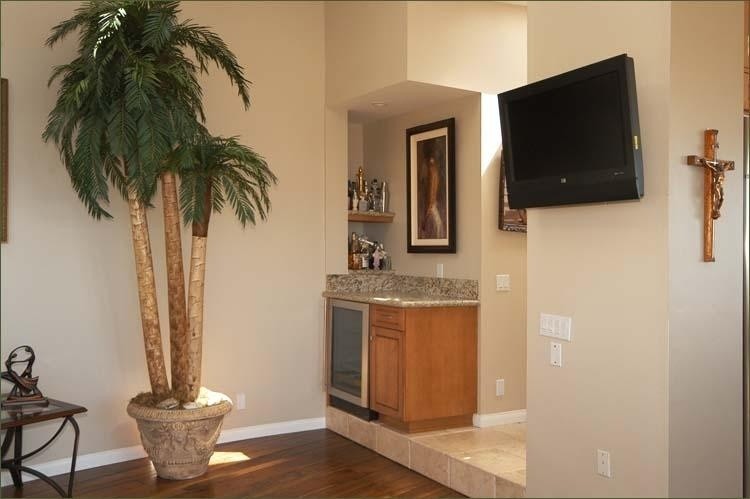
[701,157,733,219]
[419,139,444,238]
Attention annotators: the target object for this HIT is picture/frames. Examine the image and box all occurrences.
[405,118,457,254]
[500,150,526,232]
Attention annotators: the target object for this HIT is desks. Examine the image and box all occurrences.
[0,394,88,498]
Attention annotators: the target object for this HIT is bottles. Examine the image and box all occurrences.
[347,166,390,213]
[348,231,392,271]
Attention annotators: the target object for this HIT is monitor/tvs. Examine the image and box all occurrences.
[498,53,644,209]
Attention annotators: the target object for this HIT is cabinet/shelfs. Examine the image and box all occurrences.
[322,293,478,434]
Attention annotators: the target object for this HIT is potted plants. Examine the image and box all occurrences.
[41,0,280,482]
